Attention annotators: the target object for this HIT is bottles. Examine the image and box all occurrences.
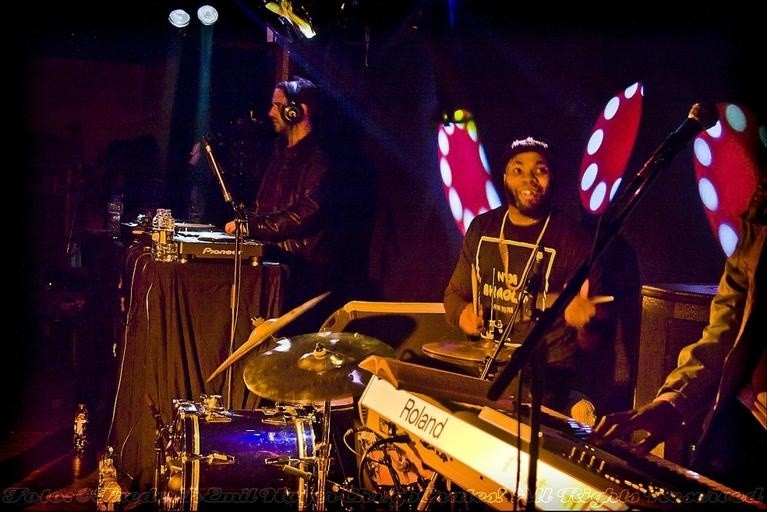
[99,457,117,508]
[106,194,124,241]
[150,209,176,262]
[188,187,206,224]
[71,403,88,452]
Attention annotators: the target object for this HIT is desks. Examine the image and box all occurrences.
[110,244,288,496]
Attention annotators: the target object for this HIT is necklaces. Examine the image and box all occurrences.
[494,207,553,292]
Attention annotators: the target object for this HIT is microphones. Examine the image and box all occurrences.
[141,395,177,464]
[635,102,720,184]
[276,464,366,502]
[186,138,210,165]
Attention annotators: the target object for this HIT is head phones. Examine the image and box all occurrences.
[276,80,303,124]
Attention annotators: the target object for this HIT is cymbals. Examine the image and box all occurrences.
[422,339,516,368]
[243,332,397,405]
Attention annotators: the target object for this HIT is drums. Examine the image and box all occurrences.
[161,395,316,512]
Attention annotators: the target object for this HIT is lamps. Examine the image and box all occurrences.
[163,1,227,32]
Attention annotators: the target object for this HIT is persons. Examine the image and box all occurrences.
[218,76,342,345]
[437,129,612,427]
[582,176,765,509]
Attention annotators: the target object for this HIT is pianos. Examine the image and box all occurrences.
[358,374,767,510]
